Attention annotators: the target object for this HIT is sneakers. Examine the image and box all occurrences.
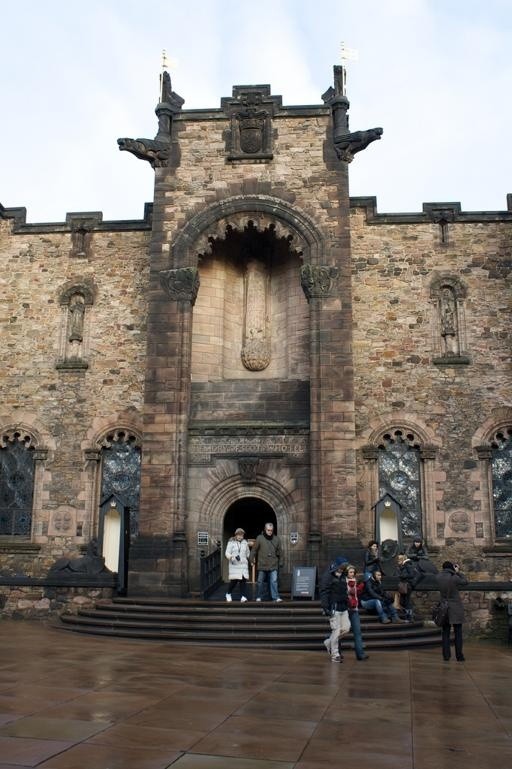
[356,654,370,661]
[256,597,262,602]
[323,639,344,663]
[240,596,248,603]
[274,598,283,603]
[381,617,410,624]
[443,655,465,662]
[225,592,232,602]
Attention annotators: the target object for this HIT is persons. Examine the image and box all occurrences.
[404,538,431,573]
[250,522,284,603]
[434,560,468,661]
[360,569,410,625]
[224,527,251,602]
[396,554,424,610]
[318,557,352,664]
[341,563,369,661]
[363,540,382,579]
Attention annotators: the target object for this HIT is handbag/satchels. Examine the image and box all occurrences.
[398,581,408,594]
[432,599,448,627]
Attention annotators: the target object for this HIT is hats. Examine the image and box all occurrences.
[368,540,378,548]
